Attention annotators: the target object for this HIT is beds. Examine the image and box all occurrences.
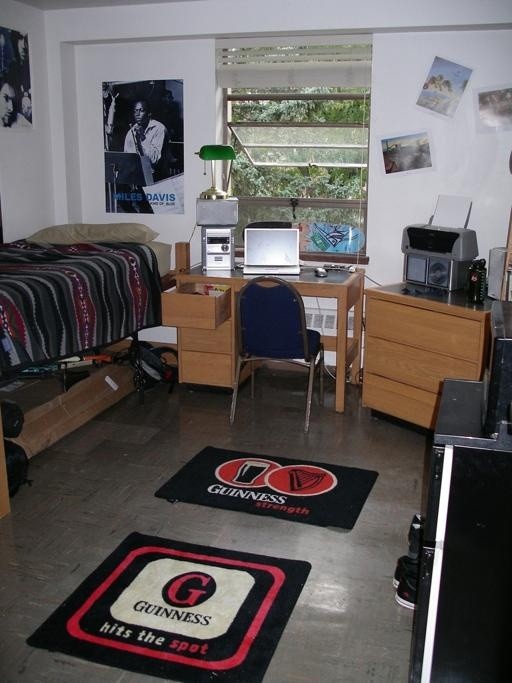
[0,242,173,500]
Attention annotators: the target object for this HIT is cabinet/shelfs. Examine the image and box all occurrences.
[359,282,493,436]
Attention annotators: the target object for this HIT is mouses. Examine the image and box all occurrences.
[314,267,327,277]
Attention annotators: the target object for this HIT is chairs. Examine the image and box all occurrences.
[229,276,326,437]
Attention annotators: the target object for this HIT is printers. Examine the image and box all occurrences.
[401,194,479,261]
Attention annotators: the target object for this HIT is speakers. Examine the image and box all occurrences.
[403,253,474,292]
[487,247,507,300]
[196,196,239,226]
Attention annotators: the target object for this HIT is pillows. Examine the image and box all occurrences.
[25,223,160,246]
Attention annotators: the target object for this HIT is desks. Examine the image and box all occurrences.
[161,261,366,416]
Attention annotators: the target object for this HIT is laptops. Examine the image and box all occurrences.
[242,228,301,275]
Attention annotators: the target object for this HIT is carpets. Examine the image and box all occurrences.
[154,442,380,531]
[25,530,313,682]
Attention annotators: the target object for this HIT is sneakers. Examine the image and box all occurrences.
[395,573,418,610]
[407,514,422,545]
[392,555,419,587]
[408,523,421,559]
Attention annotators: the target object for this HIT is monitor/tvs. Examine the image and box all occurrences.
[482,299,511,439]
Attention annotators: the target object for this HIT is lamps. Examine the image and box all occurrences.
[194,144,238,200]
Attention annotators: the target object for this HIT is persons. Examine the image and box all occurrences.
[0,76,33,129]
[20,90,32,124]
[8,29,29,77]
[124,98,173,183]
